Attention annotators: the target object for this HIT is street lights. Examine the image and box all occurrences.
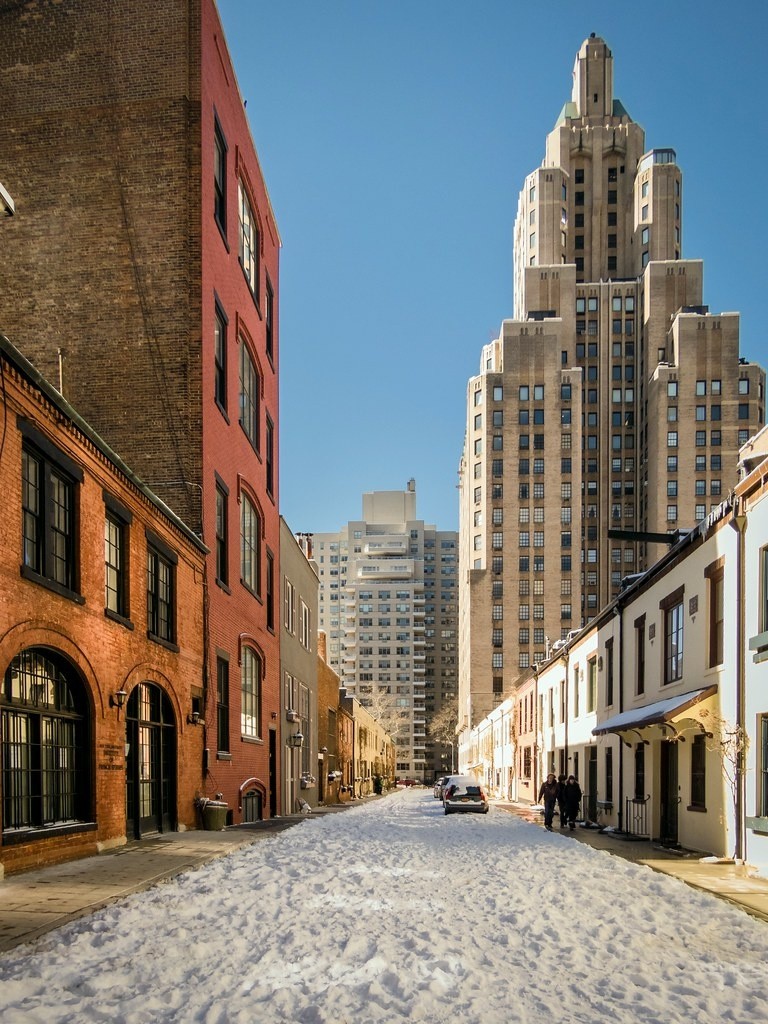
[436,740,454,775]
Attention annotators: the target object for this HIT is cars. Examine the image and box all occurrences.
[434,775,490,815]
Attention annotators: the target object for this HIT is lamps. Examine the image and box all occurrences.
[108,686,128,708]
[186,710,201,726]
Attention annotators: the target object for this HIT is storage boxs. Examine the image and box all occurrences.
[203,801,228,831]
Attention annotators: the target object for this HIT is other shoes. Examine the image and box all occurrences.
[565,819,567,825]
[545,825,553,830]
[569,822,575,831]
[561,825,564,828]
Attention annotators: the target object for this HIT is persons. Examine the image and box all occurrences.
[538,774,582,831]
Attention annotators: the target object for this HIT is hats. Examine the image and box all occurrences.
[559,775,567,781]
[569,776,575,780]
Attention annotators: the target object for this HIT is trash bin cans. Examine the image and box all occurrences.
[194,799,229,831]
